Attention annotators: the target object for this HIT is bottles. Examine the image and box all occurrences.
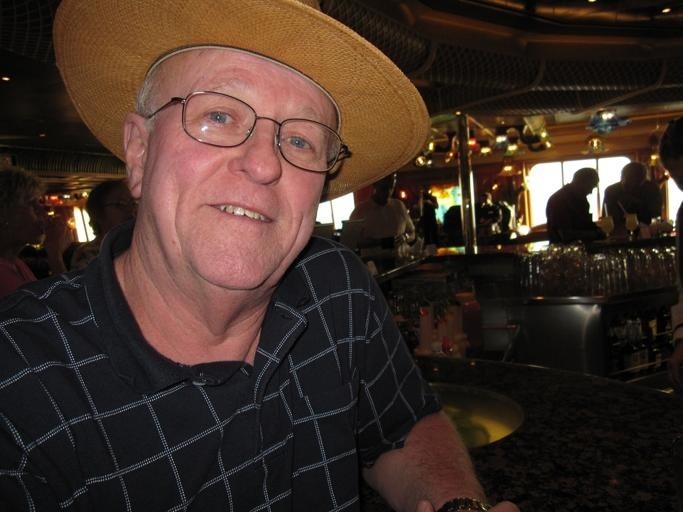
[644,305,669,342]
[621,340,650,375]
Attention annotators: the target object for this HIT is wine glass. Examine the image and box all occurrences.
[600,215,616,242]
[624,213,639,239]
[611,317,645,350]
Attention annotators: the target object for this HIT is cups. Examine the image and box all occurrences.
[532,240,678,287]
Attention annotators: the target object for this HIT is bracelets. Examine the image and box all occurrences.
[436,496,491,512]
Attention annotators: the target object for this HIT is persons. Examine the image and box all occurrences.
[476,189,502,234]
[420,184,437,246]
[350,169,416,256]
[1,0,522,512]
[546,167,609,249]
[658,115,683,394]
[601,161,662,230]
[0,167,138,298]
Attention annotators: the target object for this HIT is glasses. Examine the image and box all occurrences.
[140,91,355,174]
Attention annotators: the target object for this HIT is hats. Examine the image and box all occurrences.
[53,0,433,203]
